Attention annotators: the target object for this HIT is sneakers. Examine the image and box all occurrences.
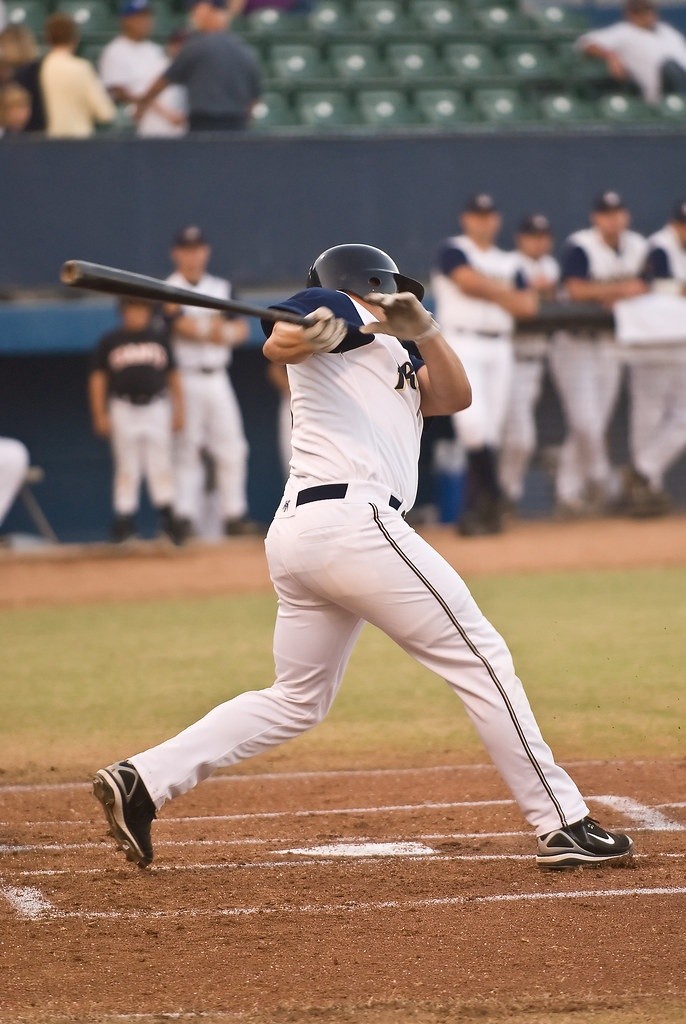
[536,815,635,873]
[90,759,157,870]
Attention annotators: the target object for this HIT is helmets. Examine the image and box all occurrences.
[308,244,425,304]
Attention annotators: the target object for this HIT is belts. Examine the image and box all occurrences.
[295,483,406,521]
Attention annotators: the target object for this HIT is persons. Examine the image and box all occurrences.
[156,224,259,543]
[622,200,686,515]
[0,14,115,137]
[574,0,686,110]
[545,191,648,515]
[493,216,561,510]
[90,295,184,544]
[428,193,539,533]
[97,0,298,134]
[93,242,632,867]
[0,437,30,524]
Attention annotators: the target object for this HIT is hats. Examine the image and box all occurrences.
[518,214,555,236]
[464,192,499,217]
[591,191,624,212]
[164,224,208,246]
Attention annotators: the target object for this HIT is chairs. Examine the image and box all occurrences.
[0,1,685,132]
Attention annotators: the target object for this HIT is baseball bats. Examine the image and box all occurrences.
[58,259,319,328]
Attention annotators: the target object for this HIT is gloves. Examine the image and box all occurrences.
[297,307,347,353]
[361,293,438,343]
